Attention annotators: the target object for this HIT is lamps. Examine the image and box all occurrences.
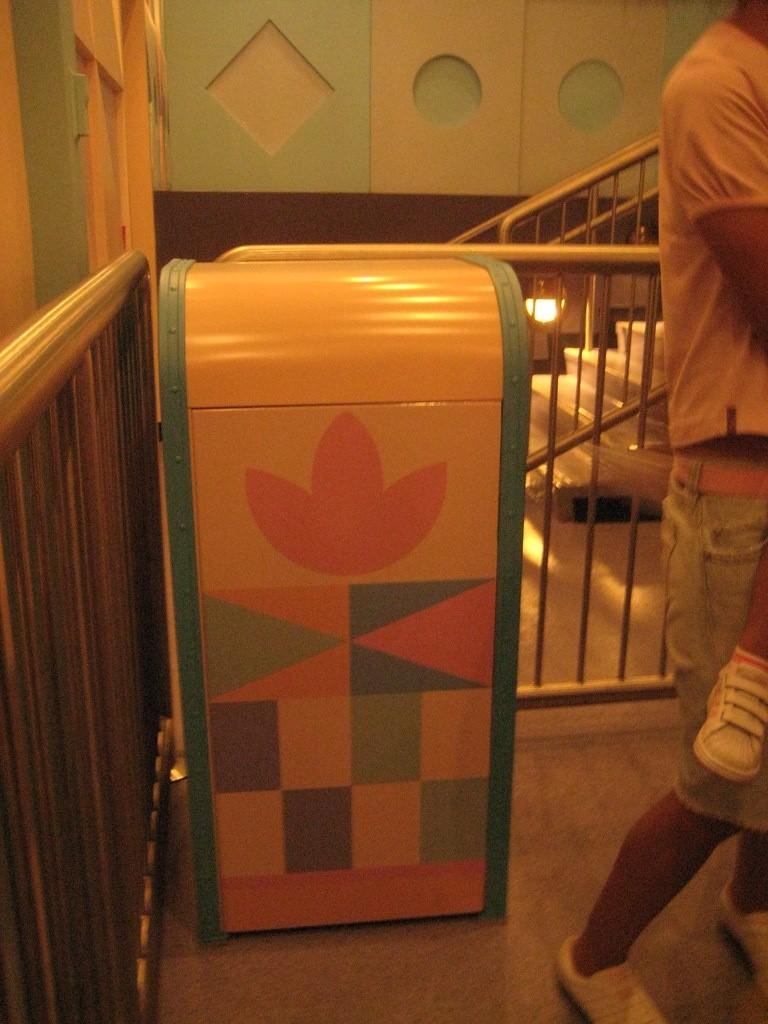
[525,281,567,324]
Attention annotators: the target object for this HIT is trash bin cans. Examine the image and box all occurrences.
[157,254,532,945]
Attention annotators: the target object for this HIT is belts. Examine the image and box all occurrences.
[673,458,768,496]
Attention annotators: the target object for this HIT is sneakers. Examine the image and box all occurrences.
[558,934,667,1024]
[719,879,768,992]
[693,663,768,784]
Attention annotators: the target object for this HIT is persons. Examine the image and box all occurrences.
[557,0,767,1023]
